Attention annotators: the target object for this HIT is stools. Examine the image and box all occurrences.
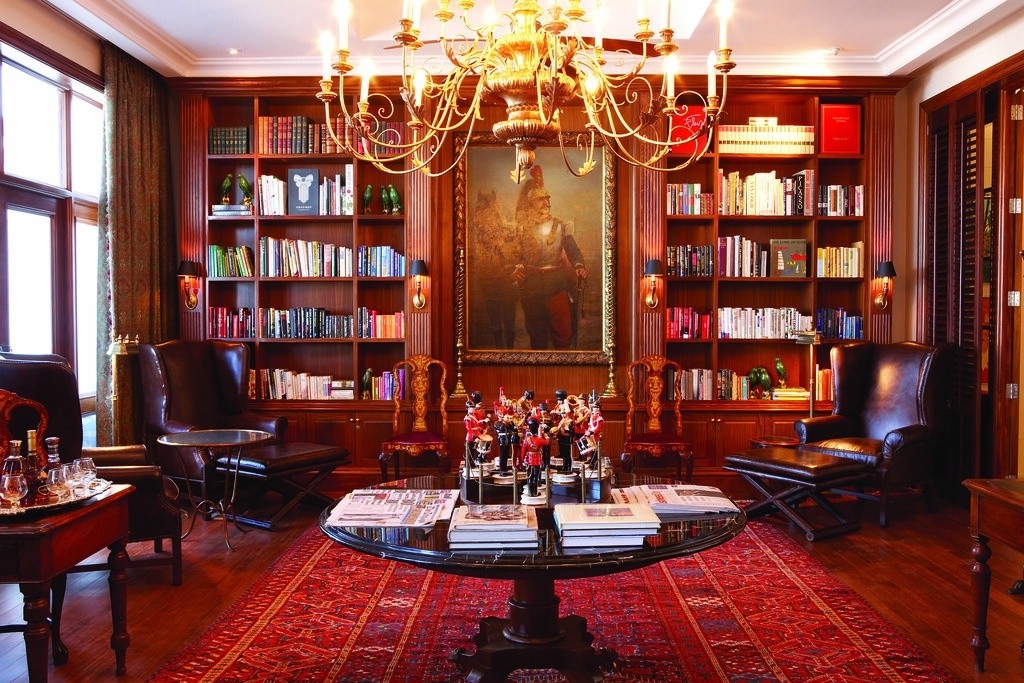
[215,441,351,530]
[722,435,869,542]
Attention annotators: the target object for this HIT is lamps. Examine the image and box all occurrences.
[315,0,734,184]
[176,260,198,310]
[875,261,899,311]
[409,260,429,309]
[643,260,664,308]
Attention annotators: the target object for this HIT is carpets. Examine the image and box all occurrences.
[142,502,963,683]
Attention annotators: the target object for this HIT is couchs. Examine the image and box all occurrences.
[0,348,184,587]
[136,338,288,520]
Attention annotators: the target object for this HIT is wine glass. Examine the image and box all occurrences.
[47,458,97,504]
[0,474,28,510]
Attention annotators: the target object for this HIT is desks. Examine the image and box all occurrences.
[0,484,136,683]
[965,479,1024,672]
[317,471,746,683]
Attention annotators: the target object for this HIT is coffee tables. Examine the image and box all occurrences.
[158,429,274,554]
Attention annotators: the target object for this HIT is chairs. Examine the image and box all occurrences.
[379,354,449,482]
[792,342,963,530]
[621,354,693,480]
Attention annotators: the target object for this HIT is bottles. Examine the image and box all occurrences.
[45,437,63,485]
[3,428,48,492]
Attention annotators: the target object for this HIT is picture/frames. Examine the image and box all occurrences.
[451,130,616,364]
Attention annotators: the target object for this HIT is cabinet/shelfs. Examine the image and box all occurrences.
[669,88,871,401]
[204,94,407,399]
